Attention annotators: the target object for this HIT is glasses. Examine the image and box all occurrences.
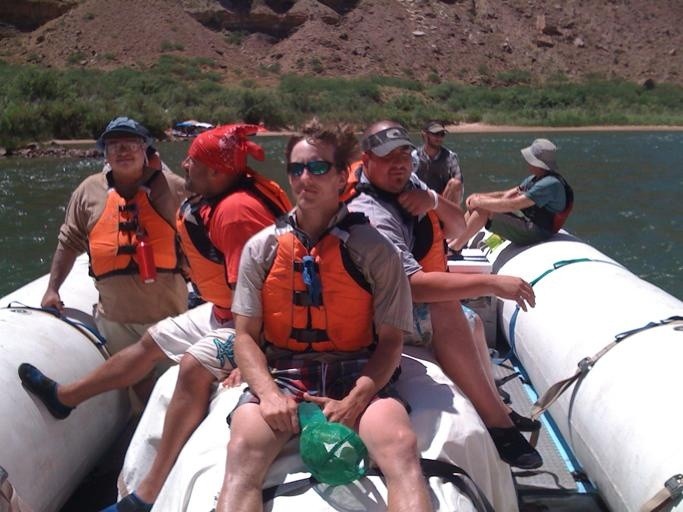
[433,132,446,136]
[287,160,337,176]
[106,143,143,155]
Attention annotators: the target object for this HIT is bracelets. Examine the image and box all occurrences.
[428,190,439,210]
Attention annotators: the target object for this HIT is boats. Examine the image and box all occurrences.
[163,119,211,144]
[0,216,683,509]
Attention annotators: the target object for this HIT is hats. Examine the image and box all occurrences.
[101,116,149,142]
[363,127,417,158]
[427,124,449,135]
[298,401,369,485]
[521,138,559,171]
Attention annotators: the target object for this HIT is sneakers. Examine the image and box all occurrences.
[447,247,464,260]
[509,410,541,431]
[18,364,76,419]
[102,493,153,512]
[488,425,543,469]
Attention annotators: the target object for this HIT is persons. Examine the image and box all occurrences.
[41,118,195,407]
[446,138,573,260]
[346,120,543,469]
[18,124,293,512]
[215,120,432,512]
[412,122,464,207]
